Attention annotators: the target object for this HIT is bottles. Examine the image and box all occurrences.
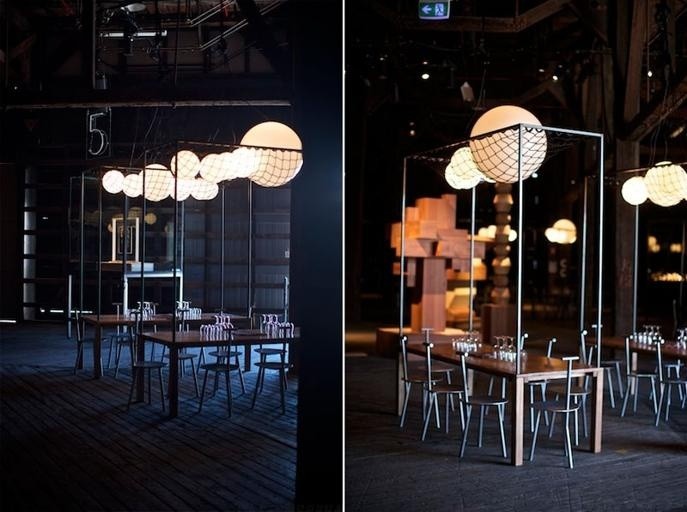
[633,332,641,343]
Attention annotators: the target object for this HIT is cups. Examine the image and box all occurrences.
[280,322,293,334]
[124,308,140,319]
[200,324,214,336]
[190,307,201,317]
[451,336,480,352]
[214,315,233,334]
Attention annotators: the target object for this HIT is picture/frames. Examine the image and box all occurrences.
[111,217,140,264]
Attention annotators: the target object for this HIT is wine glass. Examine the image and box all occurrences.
[641,324,686,350]
[136,301,154,318]
[259,313,282,334]
[175,300,192,318]
[491,335,516,361]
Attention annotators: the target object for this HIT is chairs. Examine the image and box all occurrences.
[71,295,298,421]
